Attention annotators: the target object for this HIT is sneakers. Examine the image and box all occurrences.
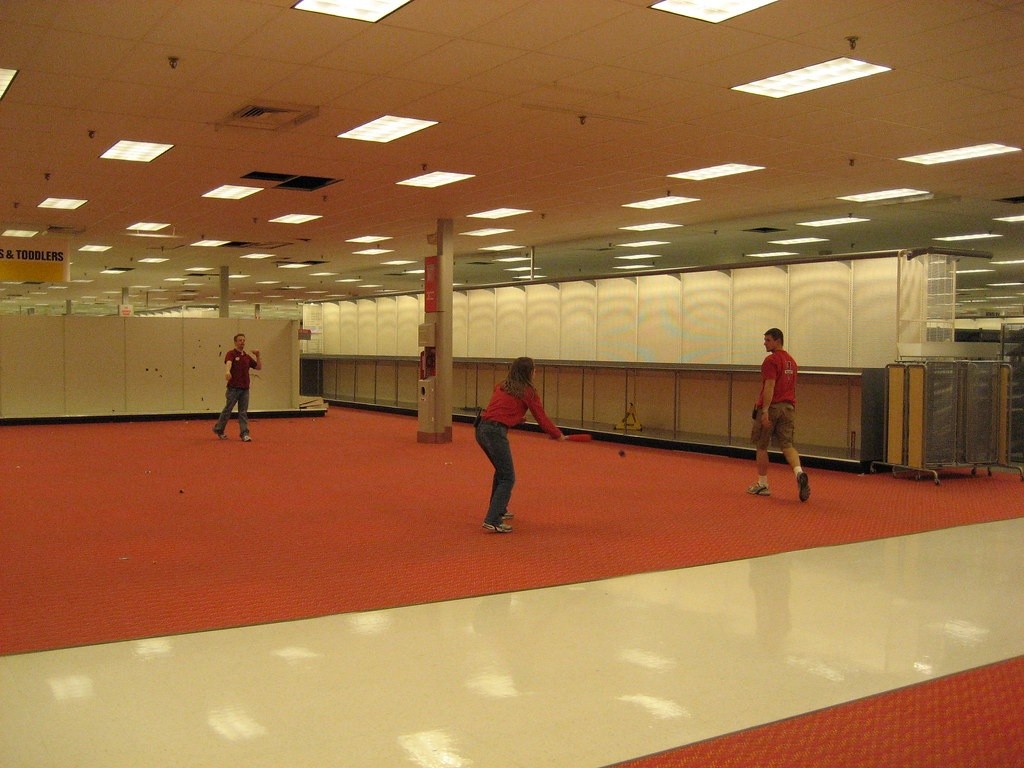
[746,483,770,495]
[213,428,228,440]
[482,519,512,532]
[240,431,252,442]
[502,512,514,519]
[797,472,811,502]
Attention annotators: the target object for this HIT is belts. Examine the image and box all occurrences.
[480,420,509,431]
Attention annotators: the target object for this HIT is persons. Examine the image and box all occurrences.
[477,356,565,533]
[746,328,811,502]
[212,334,262,442]
